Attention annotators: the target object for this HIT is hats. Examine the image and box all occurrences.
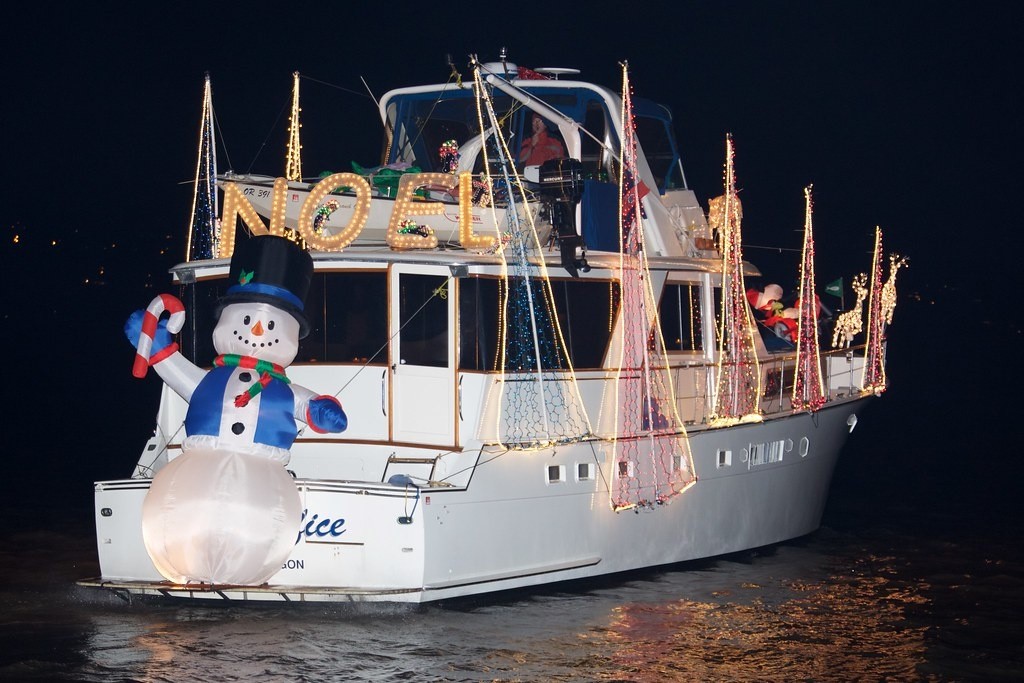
[533,111,543,120]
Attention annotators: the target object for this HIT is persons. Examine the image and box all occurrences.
[746,284,822,343]
[518,113,564,169]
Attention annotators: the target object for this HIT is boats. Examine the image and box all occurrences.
[69,43,913,617]
